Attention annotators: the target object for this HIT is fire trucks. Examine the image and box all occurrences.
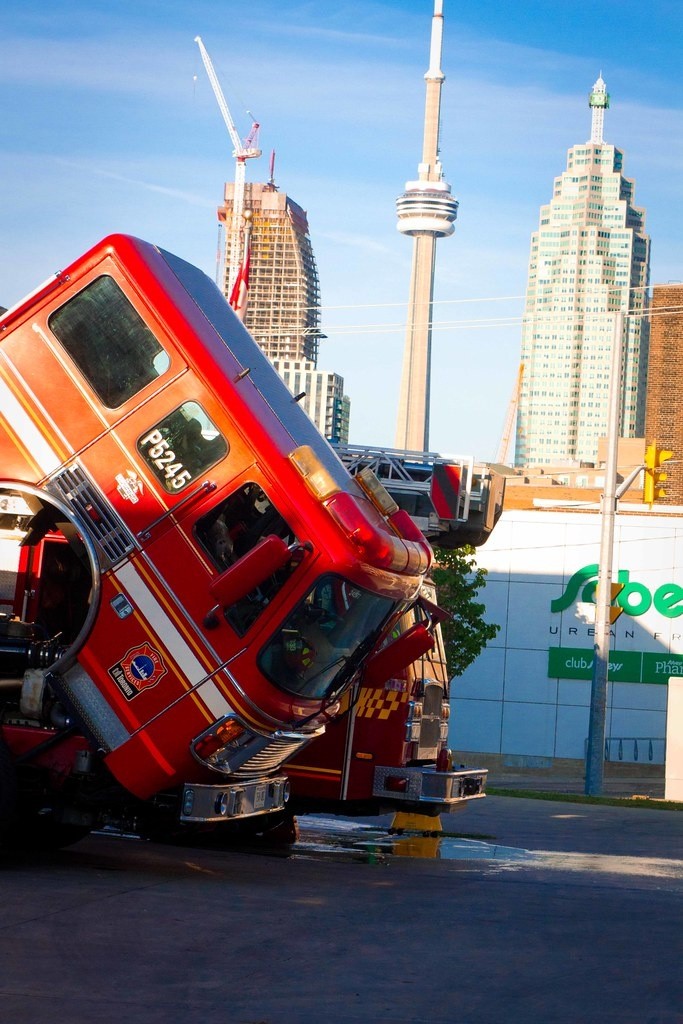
[0,234,508,867]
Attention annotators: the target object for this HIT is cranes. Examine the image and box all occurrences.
[495,361,525,464]
[194,33,262,301]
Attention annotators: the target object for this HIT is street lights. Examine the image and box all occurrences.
[583,311,666,797]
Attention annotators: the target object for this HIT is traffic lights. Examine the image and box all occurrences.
[643,438,673,502]
